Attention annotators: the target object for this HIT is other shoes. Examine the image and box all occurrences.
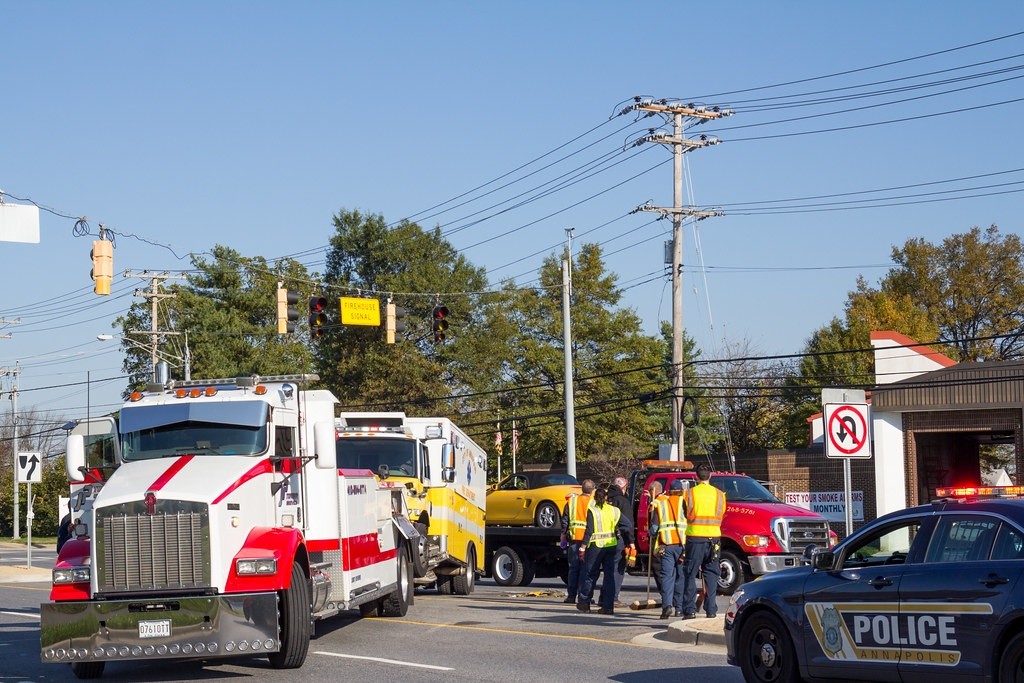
[590,598,595,604]
[577,603,590,611]
[682,613,696,620]
[676,611,683,617]
[707,613,716,618]
[660,607,672,618]
[614,601,627,608]
[564,599,575,603]
[598,606,614,615]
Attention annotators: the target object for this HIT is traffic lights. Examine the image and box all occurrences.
[395,306,405,342]
[309,296,328,338]
[432,305,449,343]
[90,248,96,295]
[287,288,298,334]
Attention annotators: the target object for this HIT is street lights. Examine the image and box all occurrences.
[97,329,190,382]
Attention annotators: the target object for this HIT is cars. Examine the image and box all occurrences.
[486,471,582,530]
[724,485,1024,683]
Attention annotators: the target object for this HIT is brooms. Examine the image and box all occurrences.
[629,486,662,611]
[696,574,708,613]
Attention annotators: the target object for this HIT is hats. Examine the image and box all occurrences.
[670,480,685,491]
[614,477,628,489]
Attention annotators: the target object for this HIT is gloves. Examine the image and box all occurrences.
[625,547,637,568]
[560,535,567,549]
[579,548,585,560]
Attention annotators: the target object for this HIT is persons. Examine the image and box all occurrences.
[643,464,727,620]
[557,476,637,615]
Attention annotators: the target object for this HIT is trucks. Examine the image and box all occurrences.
[334,411,488,595]
[484,460,838,596]
[40,362,419,680]
